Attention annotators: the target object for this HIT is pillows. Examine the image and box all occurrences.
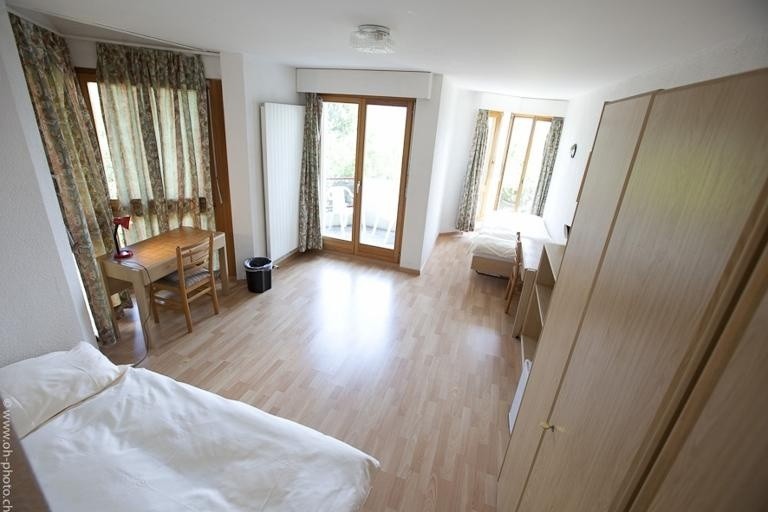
[543,213,567,235]
[554,224,566,244]
[0,342,128,438]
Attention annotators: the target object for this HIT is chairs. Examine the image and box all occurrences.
[504,231,522,313]
[149,232,220,333]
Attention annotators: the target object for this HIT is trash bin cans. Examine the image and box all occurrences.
[242,256,273,293]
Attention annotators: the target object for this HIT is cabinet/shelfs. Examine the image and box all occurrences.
[520,243,564,367]
[632,240,768,511]
[497,65,768,512]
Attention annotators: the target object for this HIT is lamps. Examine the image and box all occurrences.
[113,216,133,258]
[355,25,391,55]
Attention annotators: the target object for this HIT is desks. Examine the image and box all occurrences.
[512,237,564,338]
[97,225,230,344]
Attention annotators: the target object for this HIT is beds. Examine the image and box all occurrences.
[470,210,570,280]
[0,340,381,512]
